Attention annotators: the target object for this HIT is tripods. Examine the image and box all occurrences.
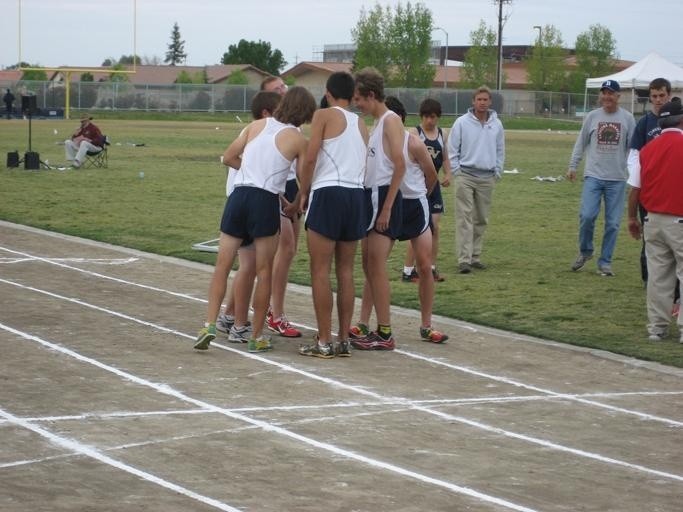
[7,114,51,171]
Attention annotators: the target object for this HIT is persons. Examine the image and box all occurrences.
[566,81,638,277]
[628,99,683,348]
[61,112,105,168]
[626,78,671,280]
[447,82,507,277]
[3,87,16,117]
[193,73,370,359]
[353,70,406,351]
[346,95,449,345]
[401,99,451,282]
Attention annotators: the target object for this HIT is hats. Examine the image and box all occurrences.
[658,102,683,117]
[80,113,94,124]
[601,81,622,93]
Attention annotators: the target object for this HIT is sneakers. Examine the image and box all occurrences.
[193,261,488,359]
[598,265,613,276]
[572,252,594,271]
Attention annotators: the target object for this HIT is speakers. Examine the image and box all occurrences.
[21,94,38,115]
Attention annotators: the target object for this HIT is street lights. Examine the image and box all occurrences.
[493,0,511,93]
[532,25,544,88]
[432,26,447,89]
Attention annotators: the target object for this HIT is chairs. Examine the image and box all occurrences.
[81,135,109,169]
